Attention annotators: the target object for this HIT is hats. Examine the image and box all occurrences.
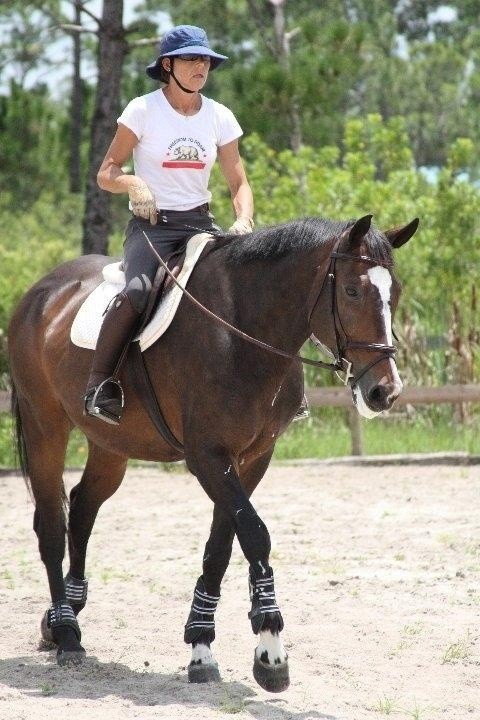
[146,25,228,83]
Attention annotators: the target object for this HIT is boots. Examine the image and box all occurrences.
[82,291,150,417]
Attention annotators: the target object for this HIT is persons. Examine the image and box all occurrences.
[82,25,310,419]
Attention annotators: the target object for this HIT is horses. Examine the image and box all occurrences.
[4,214,422,694]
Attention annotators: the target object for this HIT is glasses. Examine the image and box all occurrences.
[171,54,210,61]
